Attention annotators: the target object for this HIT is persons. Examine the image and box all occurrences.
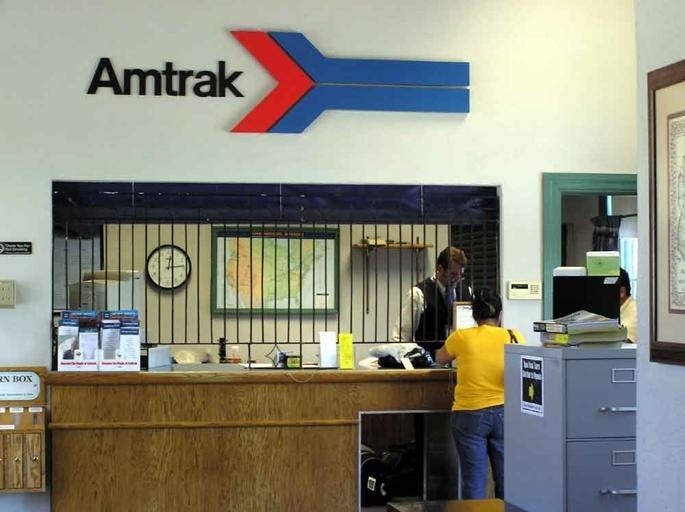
[437,288,525,500]
[392,247,467,361]
[618,268,637,344]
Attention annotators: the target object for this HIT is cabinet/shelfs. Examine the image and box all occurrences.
[505,344,644,512]
[0,367,47,497]
[50,372,509,511]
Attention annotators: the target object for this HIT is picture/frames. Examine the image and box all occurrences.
[644,60,685,365]
[210,226,343,315]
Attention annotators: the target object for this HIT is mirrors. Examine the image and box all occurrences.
[542,173,643,335]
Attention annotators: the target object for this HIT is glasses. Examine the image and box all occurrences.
[443,271,465,282]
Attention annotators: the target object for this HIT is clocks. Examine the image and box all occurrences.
[146,242,194,291]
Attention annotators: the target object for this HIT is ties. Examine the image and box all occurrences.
[445,287,455,328]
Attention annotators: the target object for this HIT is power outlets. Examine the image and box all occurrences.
[0,281,17,309]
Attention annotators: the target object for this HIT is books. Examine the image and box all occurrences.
[533,309,630,350]
